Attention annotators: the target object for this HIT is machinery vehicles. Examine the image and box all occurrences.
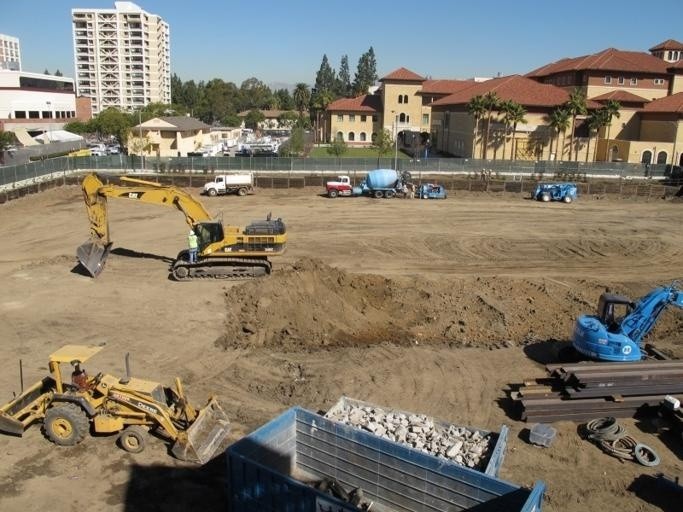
[68,169,289,284]
[0,340,232,469]
[323,167,417,199]
[527,181,577,204]
[566,279,682,363]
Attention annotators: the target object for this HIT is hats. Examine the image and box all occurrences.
[404,185,406,187]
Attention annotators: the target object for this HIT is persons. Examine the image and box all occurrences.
[409,184,414,198]
[186,230,198,264]
[403,184,408,199]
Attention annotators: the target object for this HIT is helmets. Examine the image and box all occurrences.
[188,230,195,235]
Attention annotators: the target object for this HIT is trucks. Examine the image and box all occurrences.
[201,171,259,198]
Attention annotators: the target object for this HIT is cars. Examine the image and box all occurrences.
[88,138,122,157]
[414,182,447,199]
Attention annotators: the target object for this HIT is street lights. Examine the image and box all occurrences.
[462,158,584,178]
[390,113,430,170]
[46,100,52,143]
